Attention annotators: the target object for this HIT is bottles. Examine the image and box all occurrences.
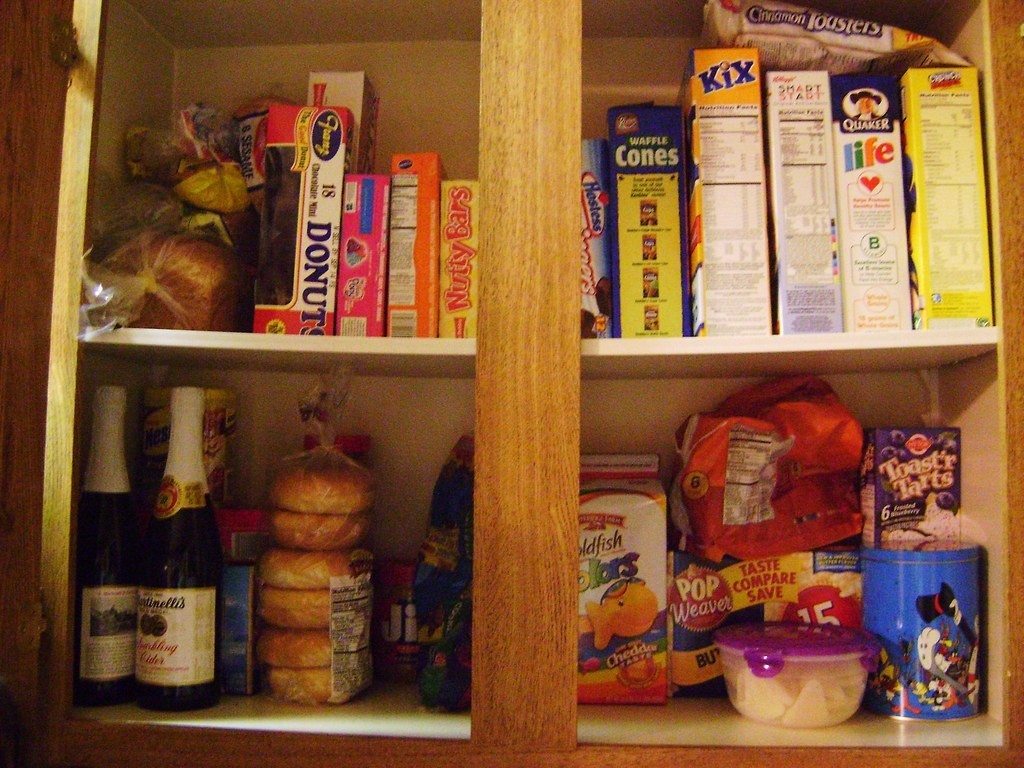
[137,386,221,710]
[74,386,137,705]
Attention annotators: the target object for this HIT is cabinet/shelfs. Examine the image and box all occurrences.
[0,0,1024,768]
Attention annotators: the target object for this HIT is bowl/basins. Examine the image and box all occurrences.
[713,621,882,727]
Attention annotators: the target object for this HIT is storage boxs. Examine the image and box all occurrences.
[256,46,995,340]
[666,547,862,701]
[857,427,961,541]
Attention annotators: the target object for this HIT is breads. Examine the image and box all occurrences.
[254,464,371,703]
[96,235,253,332]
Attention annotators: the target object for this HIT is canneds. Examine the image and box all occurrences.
[377,561,422,682]
